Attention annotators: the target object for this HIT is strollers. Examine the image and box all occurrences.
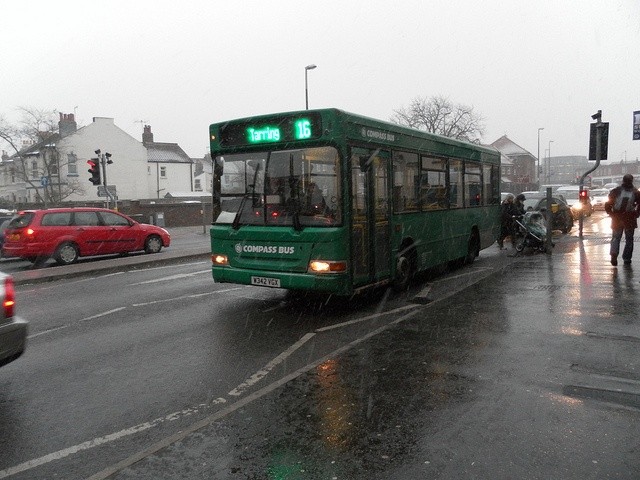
[513,211,555,253]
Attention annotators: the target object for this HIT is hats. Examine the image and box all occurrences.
[516,194,526,200]
[504,193,514,200]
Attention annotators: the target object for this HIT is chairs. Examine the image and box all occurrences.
[429,184,443,202]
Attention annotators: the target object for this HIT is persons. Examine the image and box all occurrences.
[496,194,514,251]
[604,173,640,266]
[513,193,526,221]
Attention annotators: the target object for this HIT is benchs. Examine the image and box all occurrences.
[448,184,491,209]
[419,187,446,203]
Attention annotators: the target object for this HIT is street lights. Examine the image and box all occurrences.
[538,128,544,177]
[549,140,554,175]
[305,64,317,110]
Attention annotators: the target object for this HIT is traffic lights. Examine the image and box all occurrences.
[87,158,101,186]
[580,190,588,197]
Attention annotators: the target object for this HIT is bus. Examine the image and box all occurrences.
[209,107,502,305]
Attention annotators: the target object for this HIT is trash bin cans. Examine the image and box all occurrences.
[151,211,167,230]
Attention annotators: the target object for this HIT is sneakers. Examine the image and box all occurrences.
[611,253,617,266]
[624,258,631,262]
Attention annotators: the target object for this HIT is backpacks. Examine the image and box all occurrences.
[611,187,637,214]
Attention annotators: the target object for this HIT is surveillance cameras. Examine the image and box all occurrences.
[95,149,101,154]
[107,160,113,164]
[105,152,112,157]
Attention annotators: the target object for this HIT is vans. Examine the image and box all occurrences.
[0,206,171,265]
[555,187,590,219]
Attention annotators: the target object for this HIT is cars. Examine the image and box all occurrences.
[501,192,516,205]
[0,270,28,368]
[524,196,574,233]
[0,208,15,225]
[521,191,547,210]
[589,189,610,211]
